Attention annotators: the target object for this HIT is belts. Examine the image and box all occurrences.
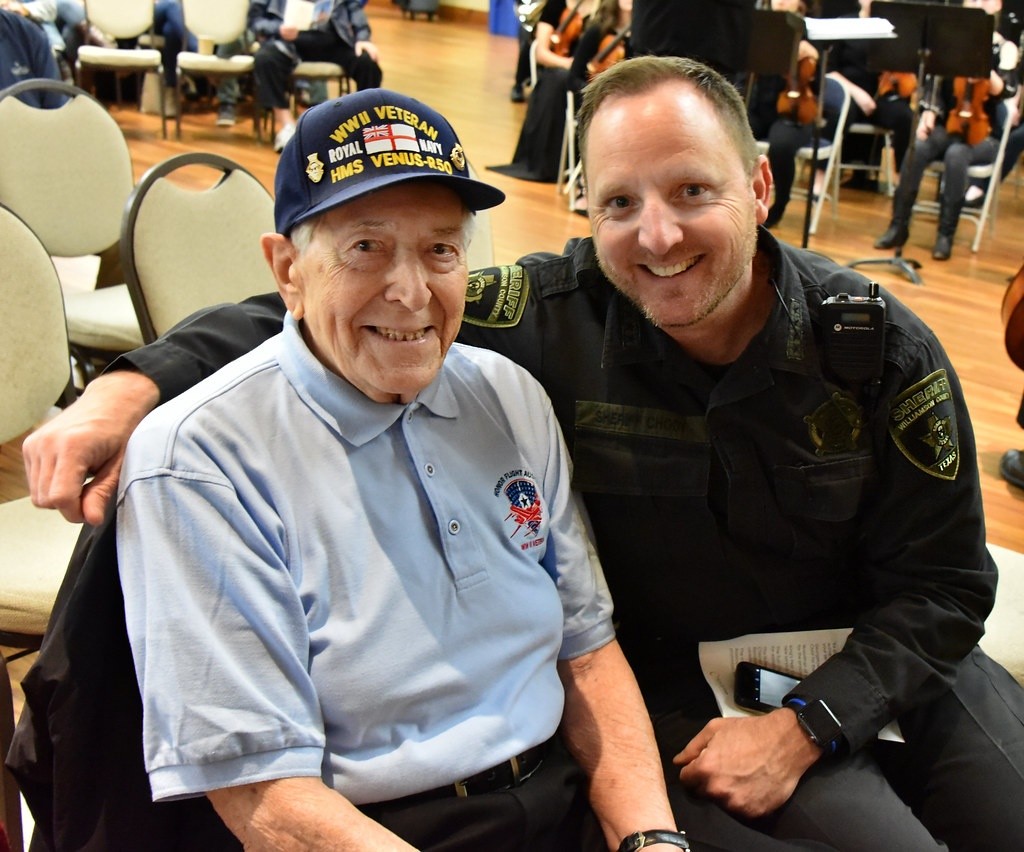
[405,730,552,799]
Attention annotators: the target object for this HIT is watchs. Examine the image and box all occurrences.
[784,696,842,764]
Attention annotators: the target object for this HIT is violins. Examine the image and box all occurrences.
[586,24,632,74]
[550,0,585,57]
[877,70,918,99]
[776,57,819,127]
[946,77,992,145]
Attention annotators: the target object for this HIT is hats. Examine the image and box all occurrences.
[272,86,507,234]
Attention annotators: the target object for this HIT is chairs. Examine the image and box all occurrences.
[6,492,246,852]
[0,0,351,652]
[524,38,1023,253]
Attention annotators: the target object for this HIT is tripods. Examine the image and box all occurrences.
[845,3,991,284]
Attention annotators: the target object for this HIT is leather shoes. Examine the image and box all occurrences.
[999,448,1023,487]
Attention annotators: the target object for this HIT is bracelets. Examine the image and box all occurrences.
[616,830,690,852]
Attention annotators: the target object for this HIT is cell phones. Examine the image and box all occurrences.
[734,662,803,713]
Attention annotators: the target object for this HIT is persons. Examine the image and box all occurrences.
[0,0,383,155]
[485,0,1024,261]
[23,58,997,852]
[116,86,692,852]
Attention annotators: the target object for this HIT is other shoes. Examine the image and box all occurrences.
[874,226,908,249]
[932,233,953,260]
[216,103,236,125]
[765,206,784,228]
[275,122,297,152]
[511,84,525,102]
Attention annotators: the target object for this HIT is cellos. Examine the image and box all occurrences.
[1001,265,1024,372]
